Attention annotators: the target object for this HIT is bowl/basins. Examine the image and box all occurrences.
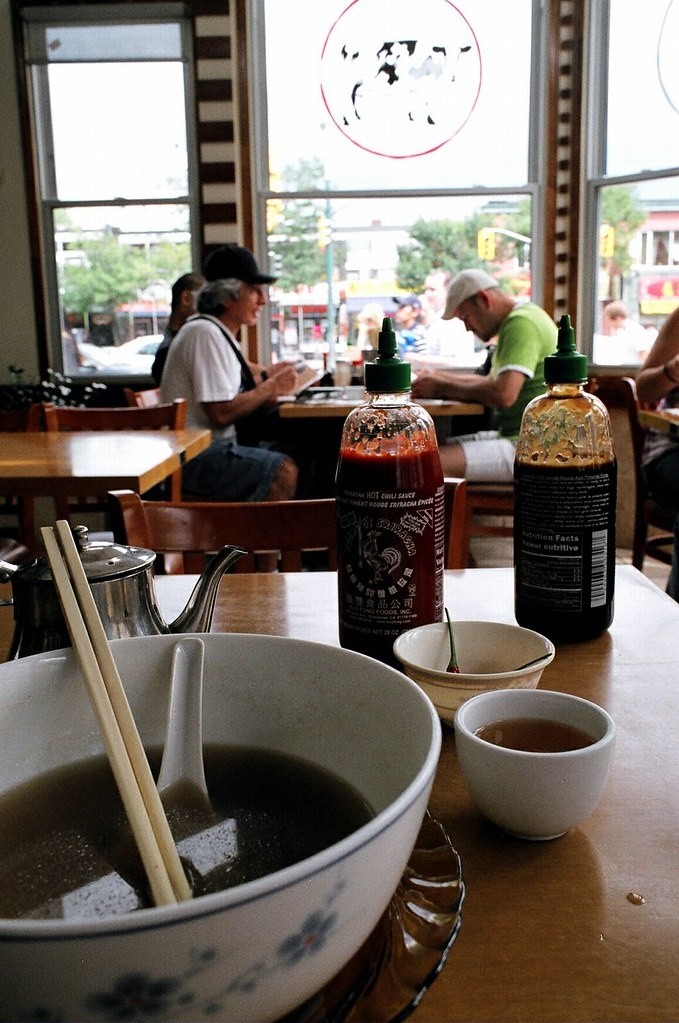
[393,621,555,722]
[0,631,443,1023]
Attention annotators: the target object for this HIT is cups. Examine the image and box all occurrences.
[454,689,616,840]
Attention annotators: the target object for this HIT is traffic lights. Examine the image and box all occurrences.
[598,224,616,258]
[477,228,495,260]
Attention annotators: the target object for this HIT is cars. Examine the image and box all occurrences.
[78,332,166,373]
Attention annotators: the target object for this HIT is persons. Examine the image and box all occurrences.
[152,243,309,502]
[637,305,679,523]
[411,269,559,483]
[359,271,657,365]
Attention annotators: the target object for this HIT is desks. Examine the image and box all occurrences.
[1,429,211,574]
[279,386,484,500]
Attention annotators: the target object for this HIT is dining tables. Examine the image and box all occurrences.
[0,563,679,1023]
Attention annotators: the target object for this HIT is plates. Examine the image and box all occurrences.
[273,808,464,1022]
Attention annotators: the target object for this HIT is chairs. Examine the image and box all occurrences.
[0,377,679,600]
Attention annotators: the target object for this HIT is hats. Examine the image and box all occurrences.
[440,268,499,320]
[202,246,279,284]
[392,294,422,309]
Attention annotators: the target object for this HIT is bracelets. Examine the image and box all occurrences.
[663,364,679,384]
[260,370,268,380]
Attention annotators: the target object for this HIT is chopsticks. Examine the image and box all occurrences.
[41,519,192,908]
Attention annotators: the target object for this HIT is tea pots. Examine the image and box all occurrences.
[0,526,248,662]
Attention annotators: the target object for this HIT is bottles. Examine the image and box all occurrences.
[335,317,446,667]
[336,360,364,386]
[512,315,617,637]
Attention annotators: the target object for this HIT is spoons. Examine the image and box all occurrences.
[156,637,210,805]
